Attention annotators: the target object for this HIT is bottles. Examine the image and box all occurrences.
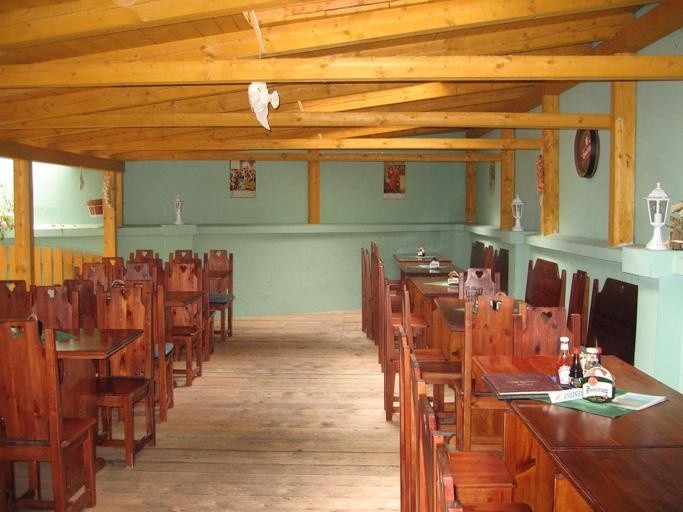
[555,335,602,389]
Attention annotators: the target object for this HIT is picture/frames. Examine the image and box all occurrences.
[572,128,601,180]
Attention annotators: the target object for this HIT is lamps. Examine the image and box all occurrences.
[642,181,670,249]
[510,194,524,232]
[171,195,185,224]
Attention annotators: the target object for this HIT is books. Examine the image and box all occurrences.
[479,370,565,400]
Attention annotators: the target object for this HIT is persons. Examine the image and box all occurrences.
[577,130,592,171]
[386,165,396,192]
[394,165,399,191]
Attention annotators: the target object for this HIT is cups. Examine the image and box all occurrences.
[465,286,482,310]
